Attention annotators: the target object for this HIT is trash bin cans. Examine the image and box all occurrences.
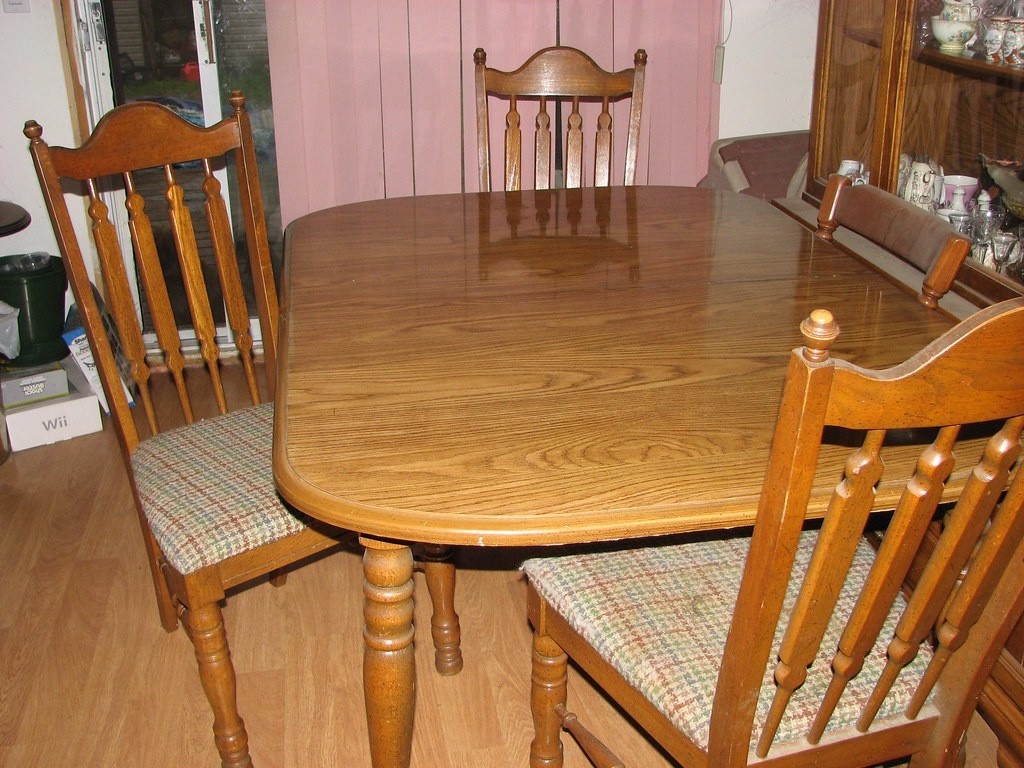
[0,252,68,365]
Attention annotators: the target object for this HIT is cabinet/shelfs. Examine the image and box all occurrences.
[803,1,1023,312]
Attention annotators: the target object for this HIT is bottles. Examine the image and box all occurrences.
[983,17,1011,61]
[1004,18,1024,66]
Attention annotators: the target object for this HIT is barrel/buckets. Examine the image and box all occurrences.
[0,254,68,365]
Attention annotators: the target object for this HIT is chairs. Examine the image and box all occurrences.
[818,172,972,311]
[471,44,648,188]
[518,294,1024,766]
[21,87,465,766]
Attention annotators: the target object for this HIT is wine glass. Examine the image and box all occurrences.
[991,233,1019,273]
[972,204,1006,265]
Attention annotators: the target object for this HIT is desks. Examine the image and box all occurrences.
[271,185,1018,768]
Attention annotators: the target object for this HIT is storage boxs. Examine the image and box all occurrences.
[0,281,136,453]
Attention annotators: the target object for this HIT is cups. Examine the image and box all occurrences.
[15,252,50,271]
[940,0,979,20]
[931,16,978,50]
[838,160,859,176]
[948,212,976,257]
